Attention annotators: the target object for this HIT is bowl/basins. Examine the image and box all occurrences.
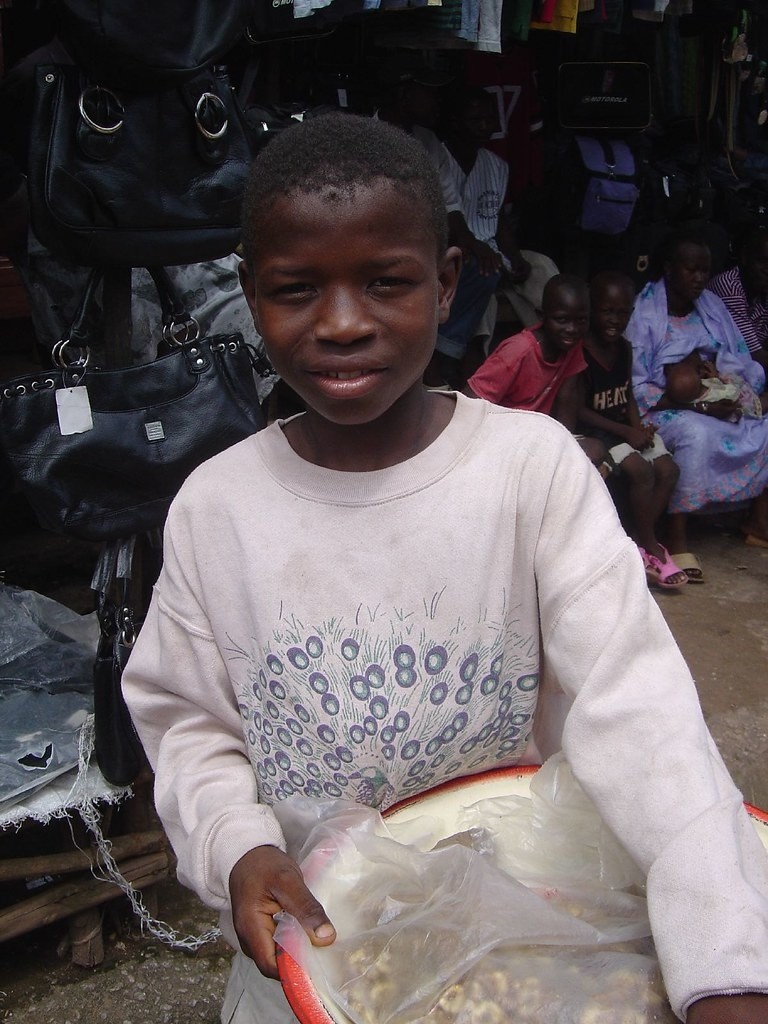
[274,766,767,1024]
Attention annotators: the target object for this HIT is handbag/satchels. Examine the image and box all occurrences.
[28,49,254,266]
[0,260,275,544]
[92,534,148,788]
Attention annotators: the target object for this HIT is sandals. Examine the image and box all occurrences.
[639,546,659,586]
[647,542,688,589]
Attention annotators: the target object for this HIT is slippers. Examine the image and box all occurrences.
[744,533,768,548]
[670,553,705,584]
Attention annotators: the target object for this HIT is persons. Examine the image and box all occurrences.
[708,232,768,362]
[464,274,605,468]
[385,26,767,387]
[119,114,768,1023]
[622,235,768,583]
[668,362,768,420]
[579,272,688,588]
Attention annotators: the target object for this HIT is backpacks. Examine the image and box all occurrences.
[562,133,644,243]
